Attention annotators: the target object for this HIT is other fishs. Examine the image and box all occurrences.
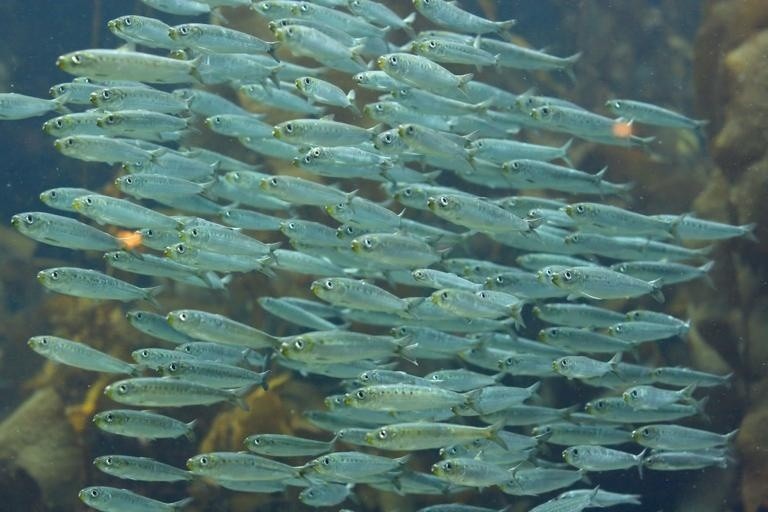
[1,1,761,512]
